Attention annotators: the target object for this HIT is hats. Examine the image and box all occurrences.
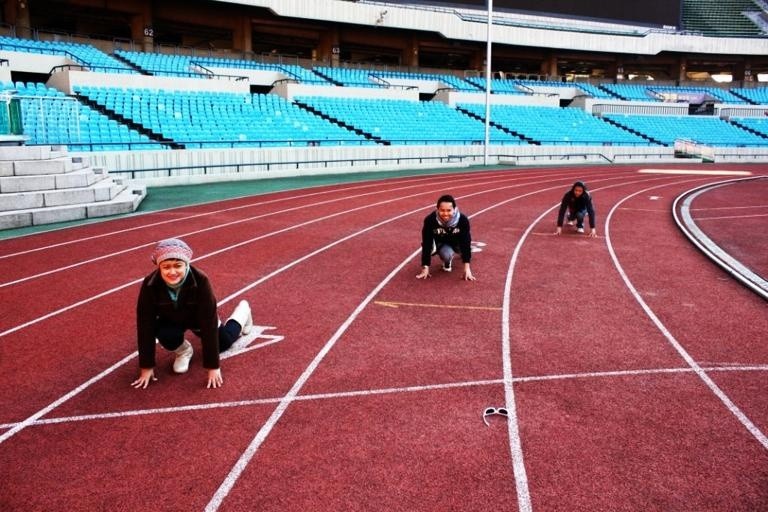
[151,239,193,268]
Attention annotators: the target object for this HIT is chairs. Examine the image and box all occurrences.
[0,1,768,150]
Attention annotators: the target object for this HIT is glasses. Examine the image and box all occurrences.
[482,407,513,428]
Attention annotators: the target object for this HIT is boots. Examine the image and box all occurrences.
[225,298,253,336]
[165,338,194,374]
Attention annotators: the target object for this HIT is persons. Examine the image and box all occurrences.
[415,194,477,281]
[553,181,596,240]
[129,237,253,390]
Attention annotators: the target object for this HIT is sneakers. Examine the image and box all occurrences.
[442,258,454,273]
[566,213,586,233]
